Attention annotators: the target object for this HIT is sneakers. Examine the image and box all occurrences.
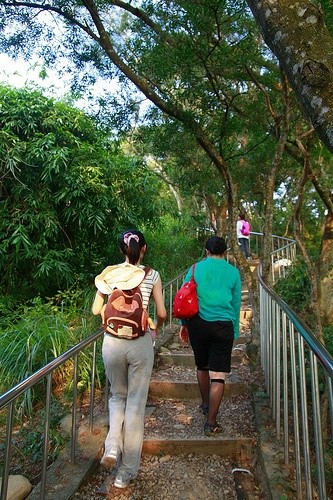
[114,474,130,488]
[100,445,121,467]
[204,421,226,436]
[197,403,209,414]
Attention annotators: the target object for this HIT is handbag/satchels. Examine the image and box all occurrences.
[174,263,198,318]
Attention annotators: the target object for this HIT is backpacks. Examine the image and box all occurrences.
[101,266,156,339]
[239,220,250,236]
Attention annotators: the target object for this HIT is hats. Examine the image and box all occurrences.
[94,263,145,294]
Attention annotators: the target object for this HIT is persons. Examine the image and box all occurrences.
[179,236,242,435]
[92,230,167,488]
[236,213,251,259]
